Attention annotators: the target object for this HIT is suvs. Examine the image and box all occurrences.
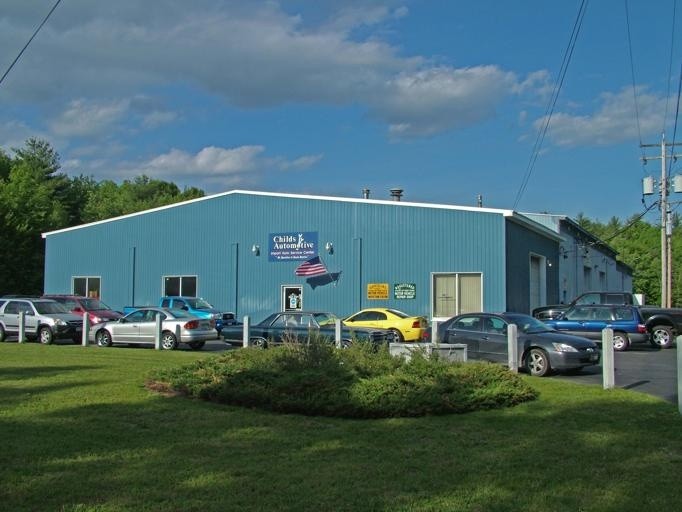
[535,303,650,351]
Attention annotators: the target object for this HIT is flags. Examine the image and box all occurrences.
[294,256,328,277]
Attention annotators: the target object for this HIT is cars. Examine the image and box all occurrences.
[0,293,240,350]
[318,307,428,344]
[219,310,395,353]
[423,311,600,378]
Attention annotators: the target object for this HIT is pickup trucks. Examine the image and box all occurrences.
[532,291,682,350]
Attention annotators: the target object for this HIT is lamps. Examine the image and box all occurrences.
[253,244,259,252]
[326,242,332,250]
